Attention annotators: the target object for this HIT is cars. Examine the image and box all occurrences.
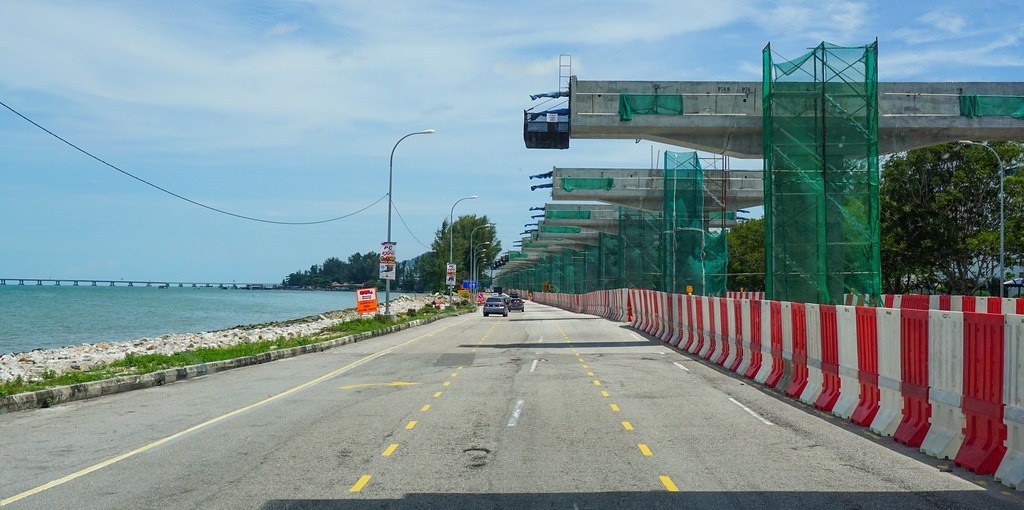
[483,297,509,317]
[492,292,519,304]
[508,298,525,312]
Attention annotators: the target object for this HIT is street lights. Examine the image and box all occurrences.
[384,129,436,314]
[472,241,490,294]
[958,139,1004,298]
[470,222,496,304]
[449,195,478,306]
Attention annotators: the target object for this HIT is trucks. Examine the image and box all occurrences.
[494,286,503,296]
